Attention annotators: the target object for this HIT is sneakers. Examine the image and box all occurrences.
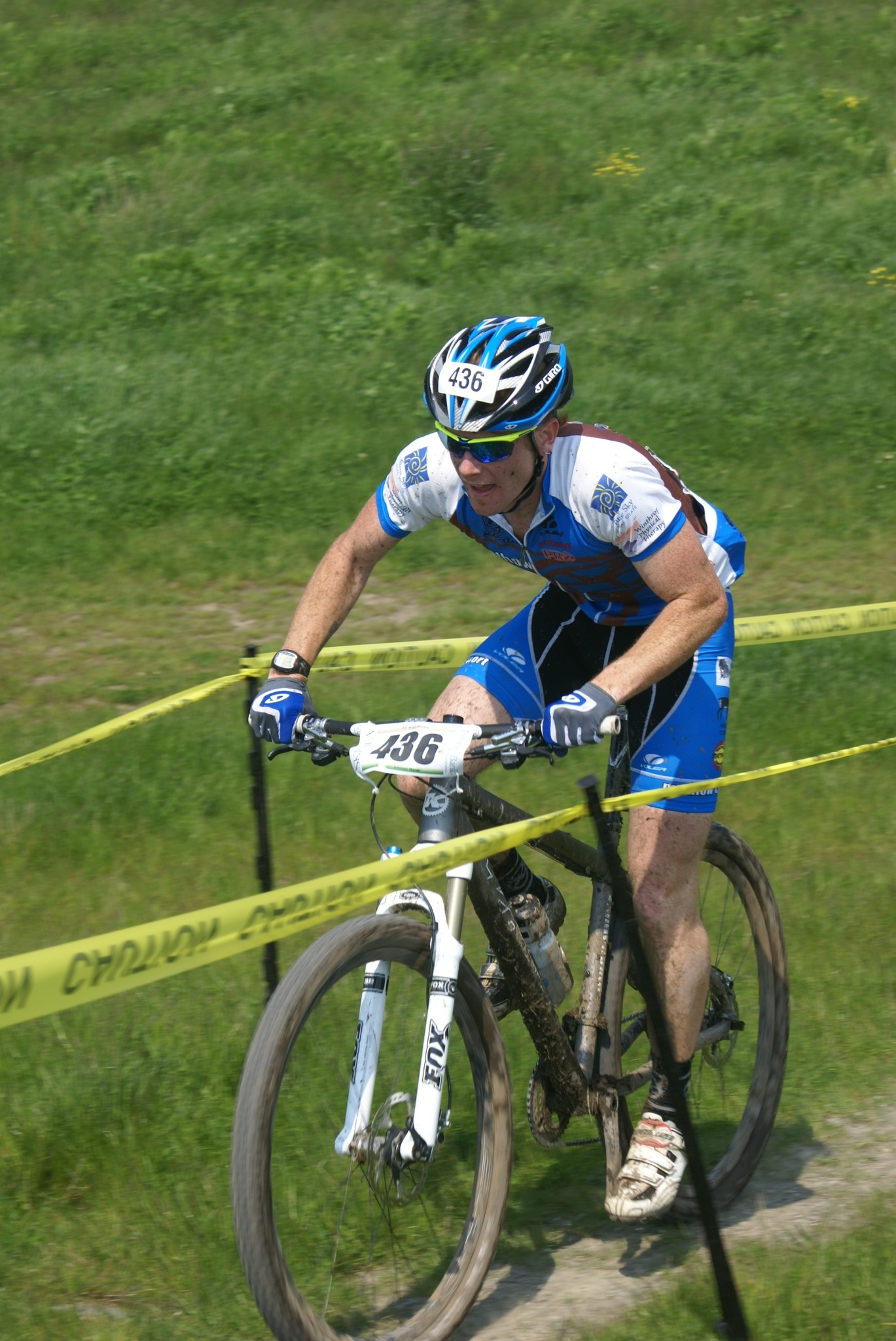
[603,1110,688,1222]
[477,875,566,1021]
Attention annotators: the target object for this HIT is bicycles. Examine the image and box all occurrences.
[232,710,790,1341]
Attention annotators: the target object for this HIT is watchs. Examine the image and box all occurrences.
[270,649,310,677]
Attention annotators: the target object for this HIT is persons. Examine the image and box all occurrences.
[248,315,747,1224]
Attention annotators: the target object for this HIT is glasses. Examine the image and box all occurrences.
[436,428,514,464]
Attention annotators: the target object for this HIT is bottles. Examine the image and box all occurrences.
[508,892,573,1000]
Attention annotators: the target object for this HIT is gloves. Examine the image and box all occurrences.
[541,681,617,759]
[248,676,319,744]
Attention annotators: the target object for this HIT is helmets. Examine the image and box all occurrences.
[424,314,575,445]
